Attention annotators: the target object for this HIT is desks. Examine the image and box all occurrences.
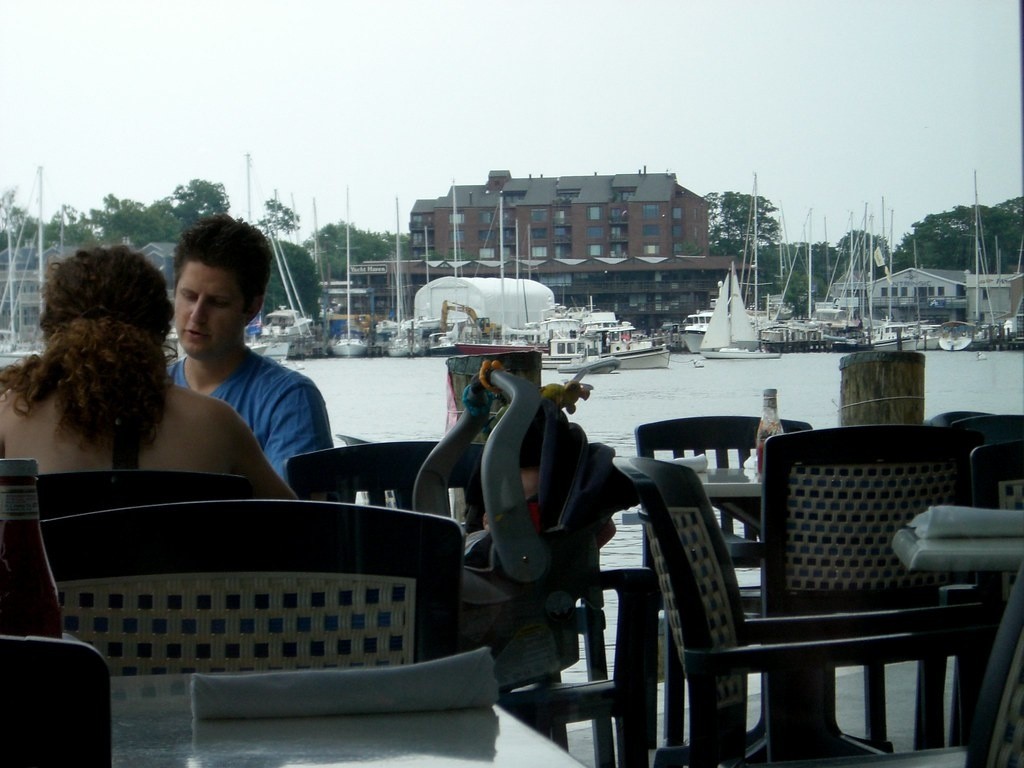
[893,527,1024,573]
[696,467,762,540]
[110,703,588,768]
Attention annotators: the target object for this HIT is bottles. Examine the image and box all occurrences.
[756,389,783,482]
[0,456,63,638]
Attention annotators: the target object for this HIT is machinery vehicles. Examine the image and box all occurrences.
[439,300,496,340]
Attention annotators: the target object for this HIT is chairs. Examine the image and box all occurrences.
[1,409,1024,767]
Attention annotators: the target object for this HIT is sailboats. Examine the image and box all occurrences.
[246,170,1024,364]
[0,165,66,370]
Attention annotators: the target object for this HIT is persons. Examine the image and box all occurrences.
[0,244,299,500]
[165,212,335,484]
[464,466,541,556]
